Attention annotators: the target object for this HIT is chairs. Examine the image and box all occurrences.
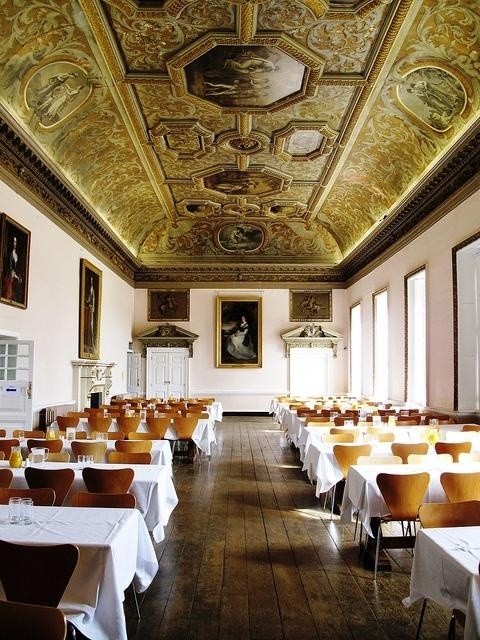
[0,397,216,640]
[369,470,431,581]
[273,394,480,543]
[416,498,480,639]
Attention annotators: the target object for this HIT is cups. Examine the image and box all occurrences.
[46,427,56,441]
[30,447,51,466]
[428,419,440,431]
[95,432,108,441]
[8,497,34,526]
[8,446,23,468]
[77,454,95,469]
[66,427,75,441]
[103,398,182,419]
[428,429,443,446]
[18,431,24,438]
[321,405,409,442]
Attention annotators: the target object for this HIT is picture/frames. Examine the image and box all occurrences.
[289,288,333,322]
[147,288,190,322]
[0,212,31,310]
[216,294,263,368]
[79,257,103,360]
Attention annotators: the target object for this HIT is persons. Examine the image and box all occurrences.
[44,84,84,121]
[229,229,240,244]
[36,72,78,99]
[2,234,22,299]
[214,172,260,193]
[84,275,96,354]
[226,315,256,360]
[408,81,461,129]
[202,55,279,99]
